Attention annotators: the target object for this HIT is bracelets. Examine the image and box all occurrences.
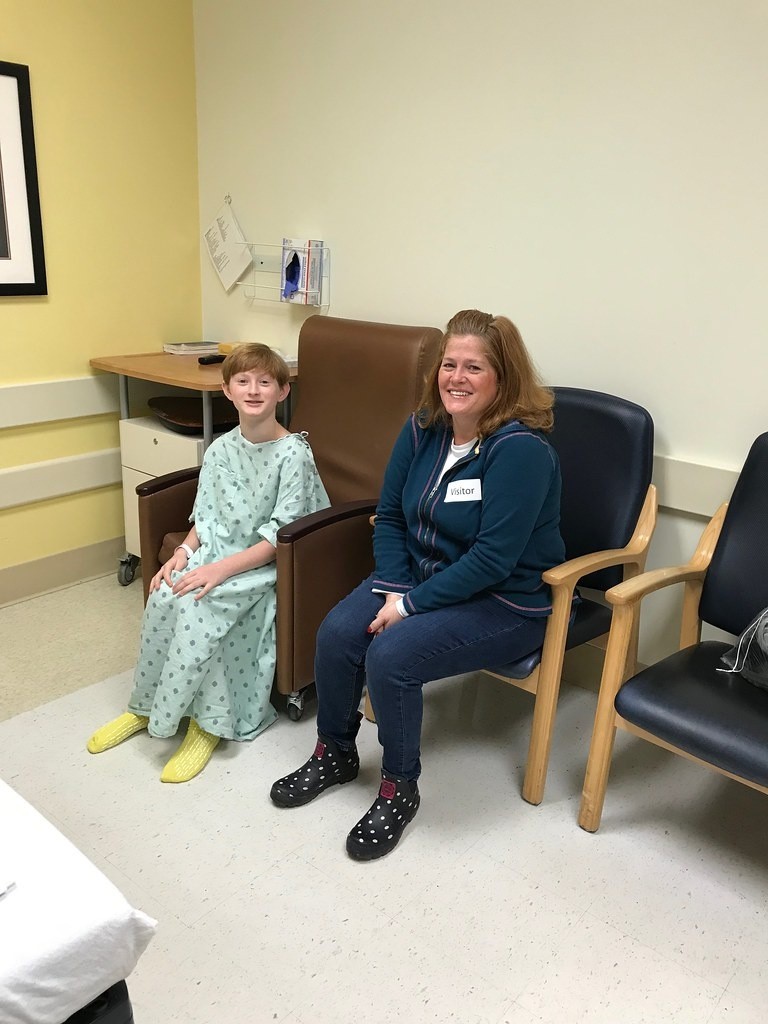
[173,543,194,559]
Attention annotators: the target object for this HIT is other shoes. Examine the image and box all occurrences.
[270,733,359,807]
[346,769,420,860]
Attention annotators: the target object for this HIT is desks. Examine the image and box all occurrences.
[90,351,298,453]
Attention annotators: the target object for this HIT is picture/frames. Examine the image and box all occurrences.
[0,61,48,295]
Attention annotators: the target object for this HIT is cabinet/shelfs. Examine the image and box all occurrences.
[118,415,227,586]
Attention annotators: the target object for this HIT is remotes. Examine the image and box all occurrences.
[198,355,227,365]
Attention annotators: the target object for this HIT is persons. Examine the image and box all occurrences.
[269,311,586,863]
[82,343,331,786]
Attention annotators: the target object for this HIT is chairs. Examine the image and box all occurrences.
[577,431,768,833]
[365,386,657,806]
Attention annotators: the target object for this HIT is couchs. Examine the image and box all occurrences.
[135,315,444,722]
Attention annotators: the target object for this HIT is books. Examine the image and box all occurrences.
[163,341,221,355]
